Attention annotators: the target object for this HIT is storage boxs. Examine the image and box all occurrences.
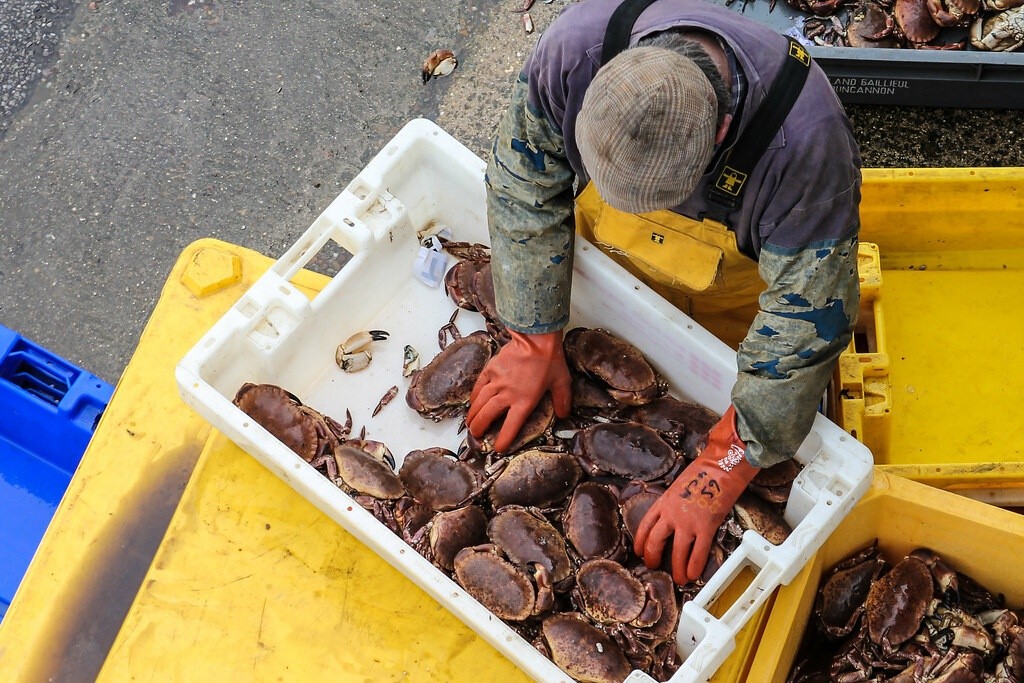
[170,116,873,682]
[704,1,1023,108]
[742,166,1024,490]
[745,479,1022,683]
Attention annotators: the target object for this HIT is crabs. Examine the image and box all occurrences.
[724,0,1023,53]
[788,542,1023,682]
[230,235,804,681]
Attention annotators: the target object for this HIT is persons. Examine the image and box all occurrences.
[466,0,862,584]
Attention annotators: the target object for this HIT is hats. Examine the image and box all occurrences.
[574,46,719,215]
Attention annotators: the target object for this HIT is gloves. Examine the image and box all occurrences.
[634,405,761,586]
[464,327,573,453]
